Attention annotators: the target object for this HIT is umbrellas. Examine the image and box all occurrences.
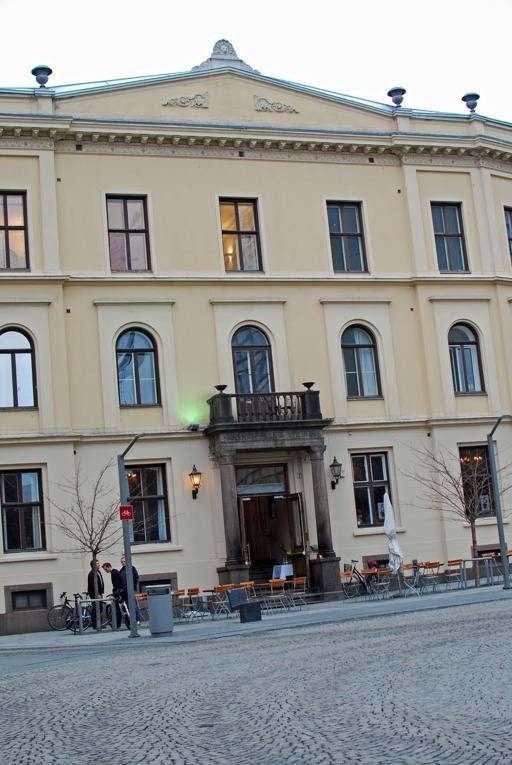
[383,492,405,594]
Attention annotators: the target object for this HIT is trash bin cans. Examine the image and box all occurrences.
[146,584,174,636]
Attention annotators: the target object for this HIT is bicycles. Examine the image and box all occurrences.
[47,591,130,633]
[341,560,390,598]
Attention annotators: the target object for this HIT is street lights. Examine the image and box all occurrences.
[117,433,147,636]
[486,415,512,589]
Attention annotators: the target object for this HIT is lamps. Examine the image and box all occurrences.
[189,465,201,499]
[187,424,199,431]
[329,456,344,489]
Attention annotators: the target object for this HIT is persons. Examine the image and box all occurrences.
[482,497,487,510]
[102,562,127,628]
[119,556,141,625]
[357,508,366,525]
[87,559,106,630]
[380,504,384,518]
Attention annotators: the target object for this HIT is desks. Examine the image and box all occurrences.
[273,564,294,578]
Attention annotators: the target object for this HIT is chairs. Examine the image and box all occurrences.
[133,577,311,625]
[339,553,505,604]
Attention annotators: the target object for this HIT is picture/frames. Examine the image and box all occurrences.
[376,500,384,522]
[480,494,491,511]
